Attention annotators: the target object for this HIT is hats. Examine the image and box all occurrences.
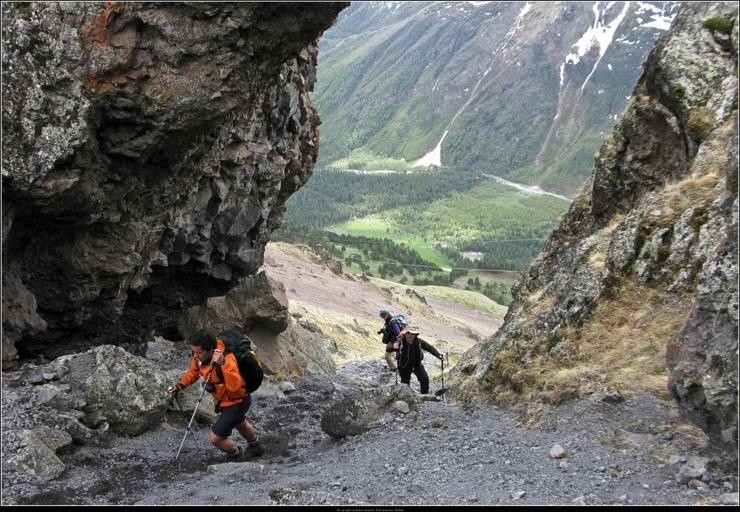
[398,323,422,336]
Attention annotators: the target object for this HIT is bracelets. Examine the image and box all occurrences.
[175,384,180,392]
[220,359,225,366]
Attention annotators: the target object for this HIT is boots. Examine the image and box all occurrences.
[215,439,264,463]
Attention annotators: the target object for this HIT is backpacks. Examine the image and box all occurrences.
[216,329,264,393]
[389,315,408,331]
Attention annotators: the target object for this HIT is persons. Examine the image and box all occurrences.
[168,328,264,459]
[376,309,443,393]
